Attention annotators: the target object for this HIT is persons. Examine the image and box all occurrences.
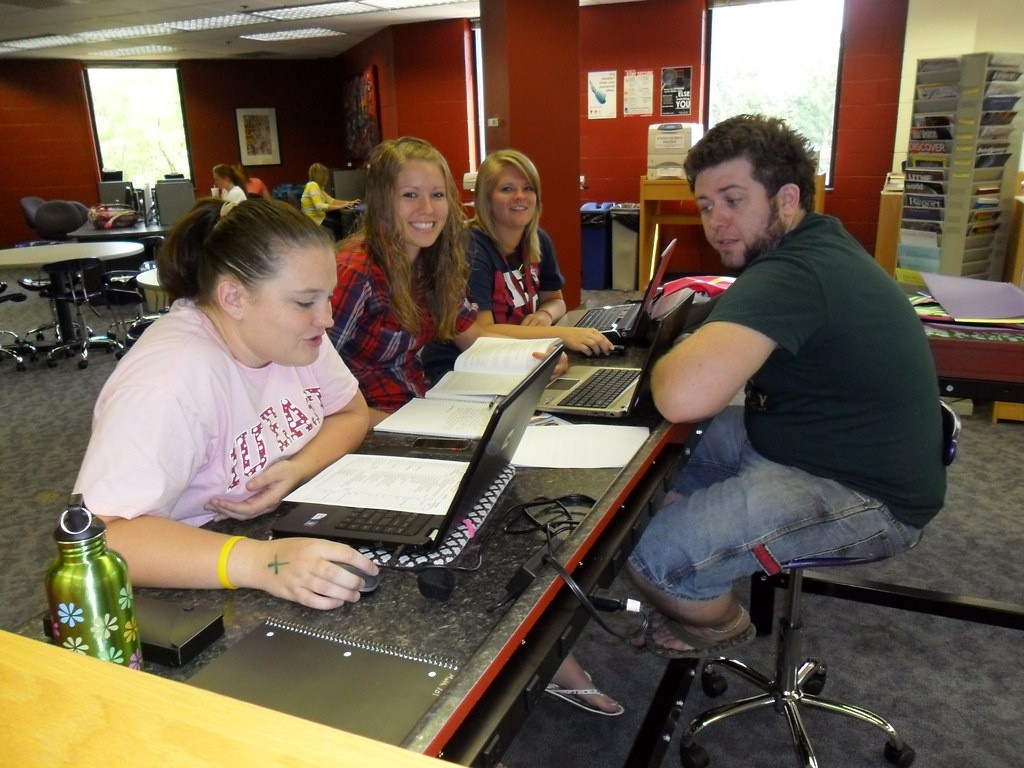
[72,195,378,610]
[325,138,623,716]
[301,163,362,240]
[213,164,270,203]
[462,147,614,357]
[630,115,946,659]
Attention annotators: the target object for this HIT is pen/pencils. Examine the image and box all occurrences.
[488,394,499,407]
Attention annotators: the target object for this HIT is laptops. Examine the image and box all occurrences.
[272,238,695,554]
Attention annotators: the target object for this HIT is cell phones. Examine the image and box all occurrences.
[411,438,473,450]
[590,345,625,354]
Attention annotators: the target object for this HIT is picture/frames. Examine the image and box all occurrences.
[235,107,282,168]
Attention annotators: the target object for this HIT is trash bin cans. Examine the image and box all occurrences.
[580,203,640,290]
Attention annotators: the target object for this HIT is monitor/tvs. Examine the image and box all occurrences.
[125,186,139,212]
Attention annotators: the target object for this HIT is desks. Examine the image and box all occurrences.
[0,241,145,366]
[68,220,167,240]
[636,170,826,295]
[13,345,700,768]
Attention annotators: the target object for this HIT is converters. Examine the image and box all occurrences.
[506,536,563,600]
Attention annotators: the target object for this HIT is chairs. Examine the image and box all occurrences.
[0,196,169,369]
[680,401,963,768]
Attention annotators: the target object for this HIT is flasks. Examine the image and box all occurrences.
[44,493,142,684]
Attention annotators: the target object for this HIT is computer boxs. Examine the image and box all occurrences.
[329,170,365,202]
[155,179,196,226]
[98,181,134,204]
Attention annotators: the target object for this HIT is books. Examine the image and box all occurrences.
[898,58,1024,274]
[425,337,563,403]
[374,398,497,440]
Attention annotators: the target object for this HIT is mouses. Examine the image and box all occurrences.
[329,561,380,595]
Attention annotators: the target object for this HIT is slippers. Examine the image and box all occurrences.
[643,617,757,660]
[543,670,625,717]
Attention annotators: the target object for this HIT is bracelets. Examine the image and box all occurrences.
[539,309,553,320]
[218,535,246,589]
